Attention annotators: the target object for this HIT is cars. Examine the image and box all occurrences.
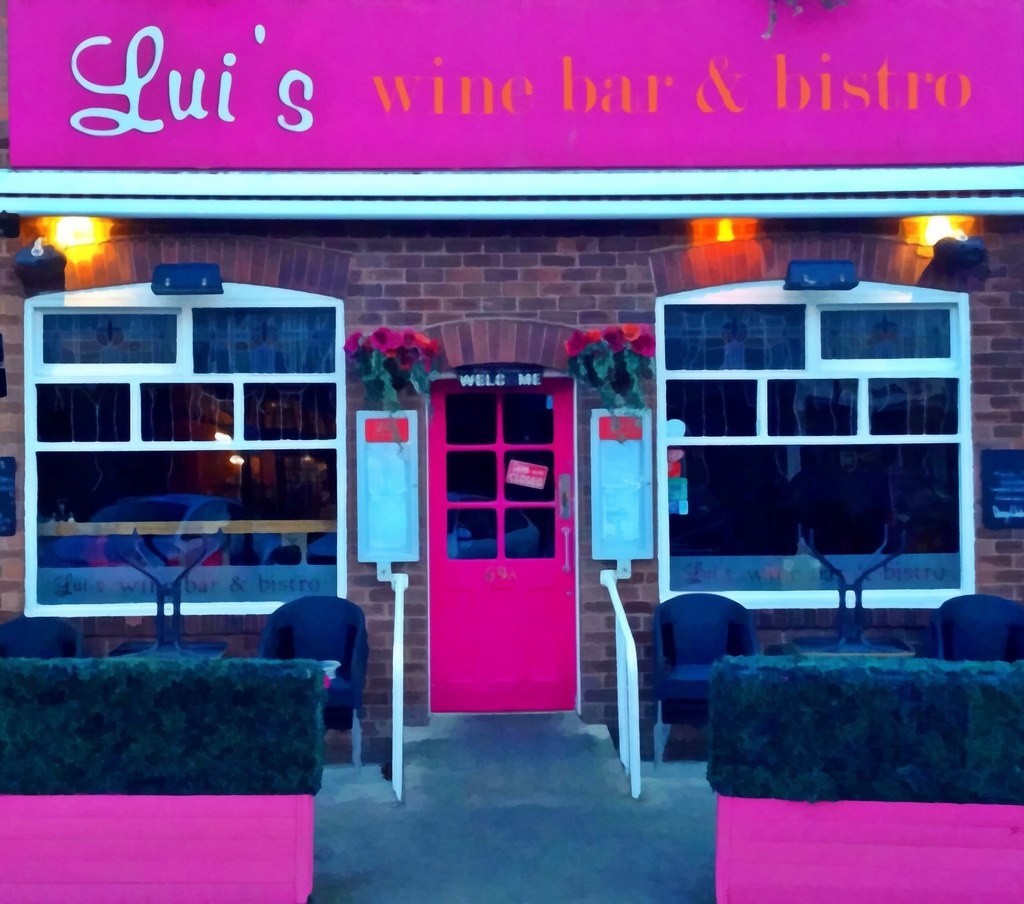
[310,495,546,566]
[43,490,252,570]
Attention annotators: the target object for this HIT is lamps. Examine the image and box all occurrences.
[783,259,861,292]
[151,263,224,294]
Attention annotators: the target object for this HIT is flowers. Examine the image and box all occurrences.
[344,328,440,467]
[562,325,657,442]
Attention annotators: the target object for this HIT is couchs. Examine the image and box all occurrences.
[253,595,367,766]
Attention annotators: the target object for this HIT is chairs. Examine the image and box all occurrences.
[653,593,757,775]
[936,593,1024,663]
[0,614,83,659]
